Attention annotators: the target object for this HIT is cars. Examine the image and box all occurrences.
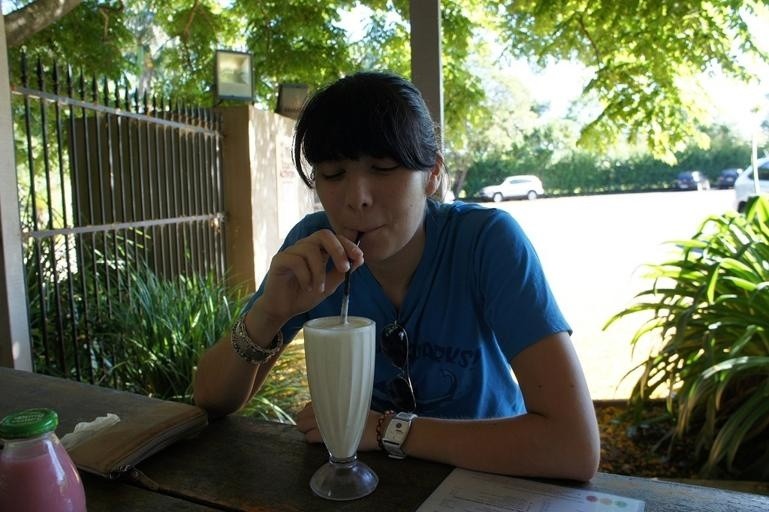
[718,167,742,189]
[734,156,769,213]
[674,169,711,191]
[479,174,544,202]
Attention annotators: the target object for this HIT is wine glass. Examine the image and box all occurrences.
[301,314,381,501]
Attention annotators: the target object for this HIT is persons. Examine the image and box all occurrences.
[192,70,601,485]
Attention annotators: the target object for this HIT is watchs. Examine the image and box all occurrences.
[383,412,418,460]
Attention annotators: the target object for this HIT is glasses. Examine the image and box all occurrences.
[378,318,418,412]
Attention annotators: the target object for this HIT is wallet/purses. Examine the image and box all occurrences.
[65,400,209,491]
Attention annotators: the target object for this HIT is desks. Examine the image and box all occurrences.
[85,407,766,510]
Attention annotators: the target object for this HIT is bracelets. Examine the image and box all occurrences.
[230,310,283,366]
[376,409,396,451]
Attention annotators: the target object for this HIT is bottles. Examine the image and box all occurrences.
[0,405,89,512]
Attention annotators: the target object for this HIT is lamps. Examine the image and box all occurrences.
[207,47,310,120]
